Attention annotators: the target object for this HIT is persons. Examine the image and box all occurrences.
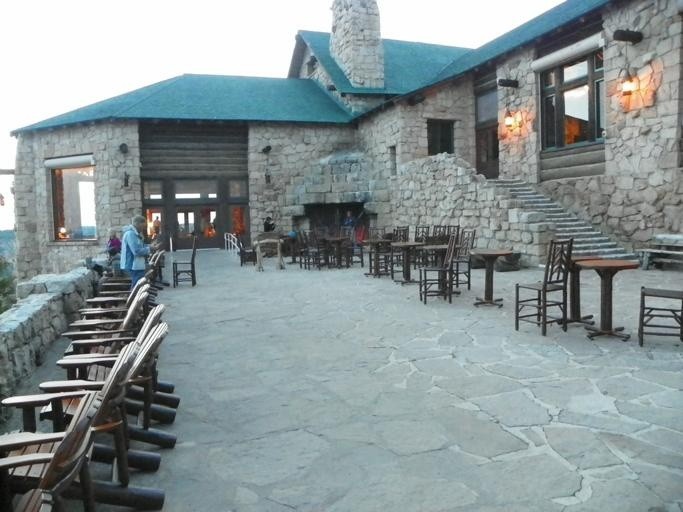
[121,216,159,289]
[264,217,275,231]
[106,231,121,260]
[153,216,161,236]
[199,216,217,238]
[345,211,356,242]
[288,223,296,238]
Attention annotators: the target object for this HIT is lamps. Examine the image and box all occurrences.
[621,39,633,95]
[503,85,513,126]
